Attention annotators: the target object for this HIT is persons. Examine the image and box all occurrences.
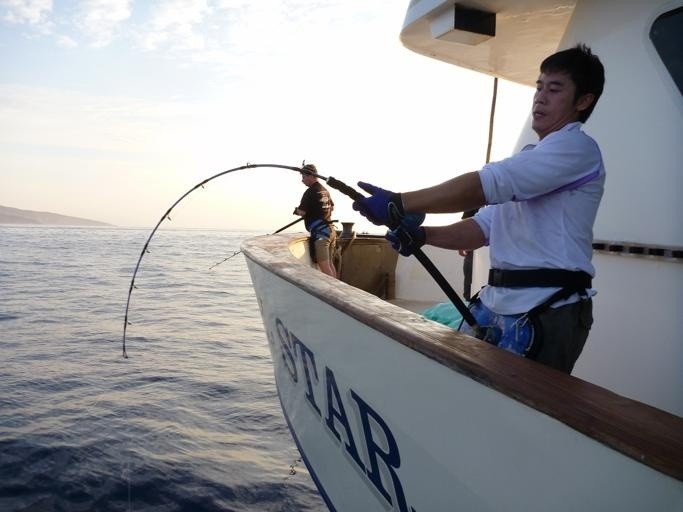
[352,42,605,374]
[293,163,338,278]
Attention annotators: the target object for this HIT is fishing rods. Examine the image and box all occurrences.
[209,217,306,271]
[122,164,491,358]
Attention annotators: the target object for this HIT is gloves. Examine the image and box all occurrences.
[384,225,427,257]
[350,179,398,225]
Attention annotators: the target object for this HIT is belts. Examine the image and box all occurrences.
[488,268,594,291]
